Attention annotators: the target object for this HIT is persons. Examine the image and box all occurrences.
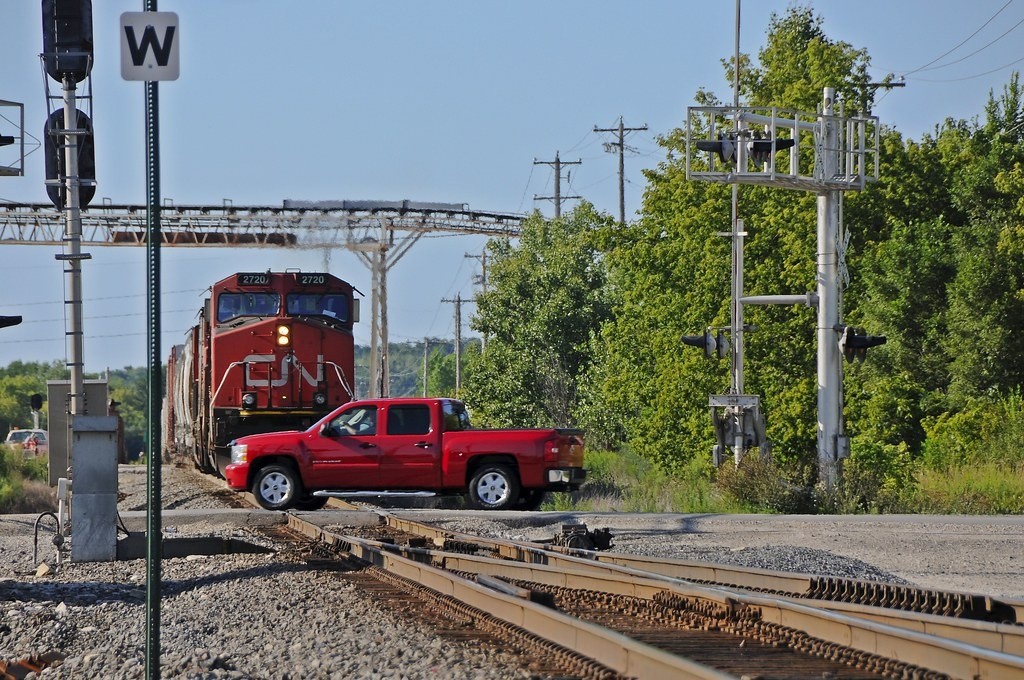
[339,408,377,435]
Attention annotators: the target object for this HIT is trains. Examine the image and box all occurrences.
[164,266,366,478]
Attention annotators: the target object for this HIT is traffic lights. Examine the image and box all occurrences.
[684,329,729,362]
[840,323,889,365]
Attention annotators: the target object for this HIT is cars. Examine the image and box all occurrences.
[6,429,50,457]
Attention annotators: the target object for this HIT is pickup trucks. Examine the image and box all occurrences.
[225,396,587,511]
